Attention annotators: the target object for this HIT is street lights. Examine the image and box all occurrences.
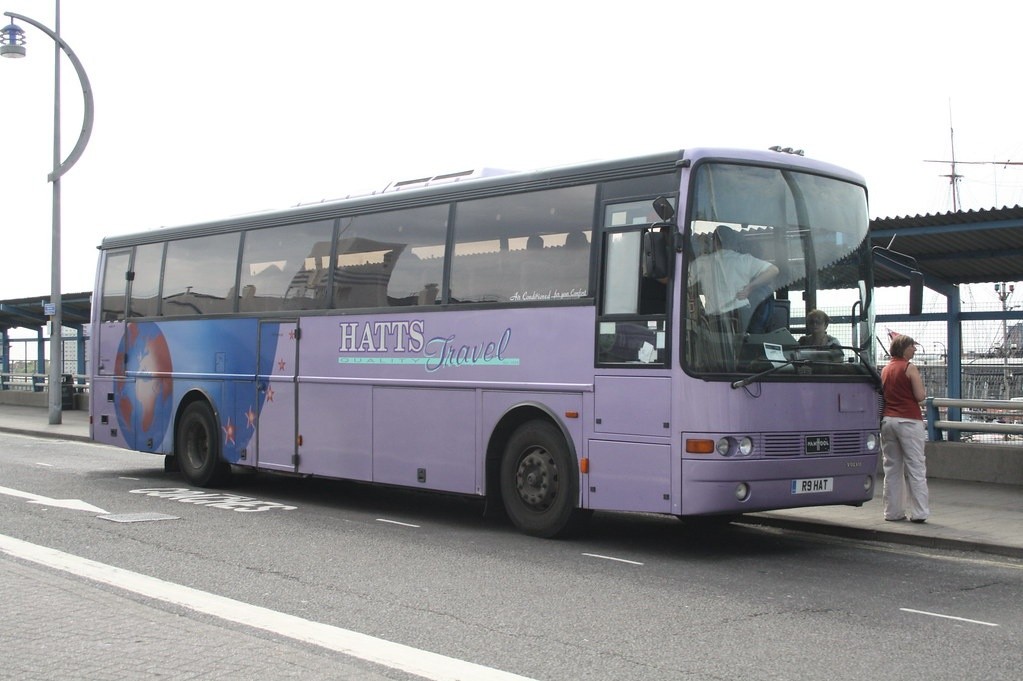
[0,0,95,425]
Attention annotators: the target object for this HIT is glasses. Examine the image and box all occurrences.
[808,317,824,325]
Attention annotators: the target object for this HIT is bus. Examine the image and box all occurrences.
[92,144,885,542]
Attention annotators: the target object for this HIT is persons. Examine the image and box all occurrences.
[688,225,779,376]
[376,224,676,318]
[798,309,846,363]
[881,334,930,523]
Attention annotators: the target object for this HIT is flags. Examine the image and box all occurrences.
[885,327,921,345]
